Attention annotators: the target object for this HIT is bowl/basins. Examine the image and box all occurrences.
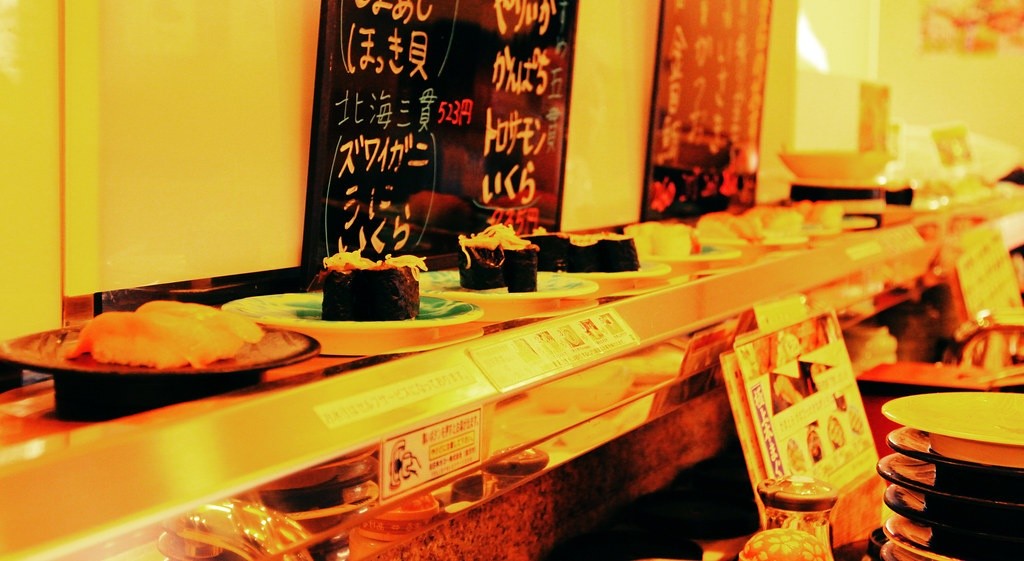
[781,151,889,180]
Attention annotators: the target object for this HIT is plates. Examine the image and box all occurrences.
[221,291,484,356]
[416,269,600,323]
[0,322,322,423]
[878,391,1024,561]
[572,262,671,281]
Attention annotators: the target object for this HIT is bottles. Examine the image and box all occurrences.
[346,493,440,561]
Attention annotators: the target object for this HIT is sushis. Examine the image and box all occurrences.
[61,299,264,369]
[314,200,844,321]
